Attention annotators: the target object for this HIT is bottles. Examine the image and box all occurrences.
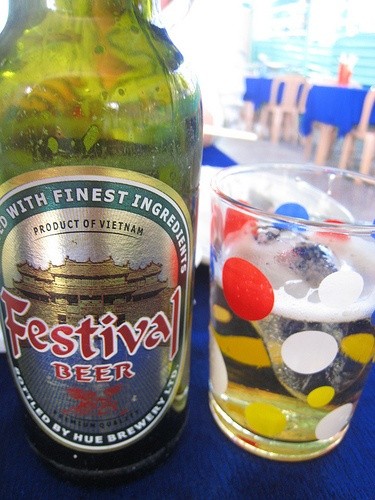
[0,0,201,486]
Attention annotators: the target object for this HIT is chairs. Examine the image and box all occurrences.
[242,74,375,175]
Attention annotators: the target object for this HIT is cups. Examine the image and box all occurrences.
[207,164,375,463]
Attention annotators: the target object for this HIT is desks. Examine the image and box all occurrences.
[298,85,375,166]
[243,79,285,132]
